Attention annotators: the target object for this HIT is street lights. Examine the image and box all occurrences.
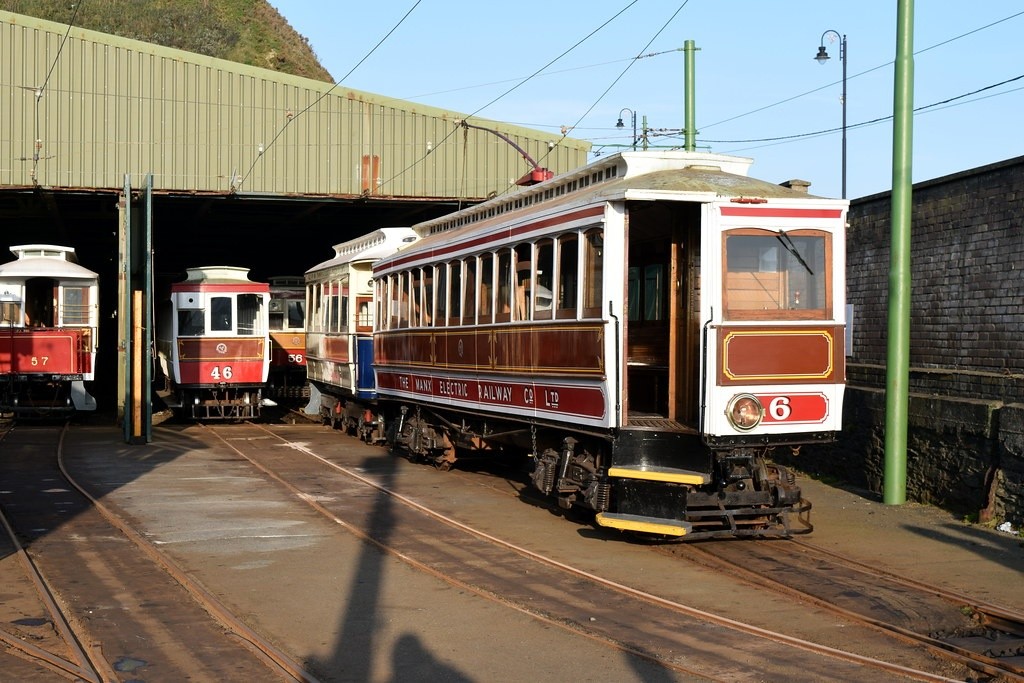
[812,30,848,198]
[615,108,637,152]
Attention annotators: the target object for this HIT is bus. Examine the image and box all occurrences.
[268,276,310,401]
[370,149,850,543]
[154,266,273,424]
[286,226,423,445]
[0,242,100,419]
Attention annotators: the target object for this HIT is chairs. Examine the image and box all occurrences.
[628,319,669,412]
